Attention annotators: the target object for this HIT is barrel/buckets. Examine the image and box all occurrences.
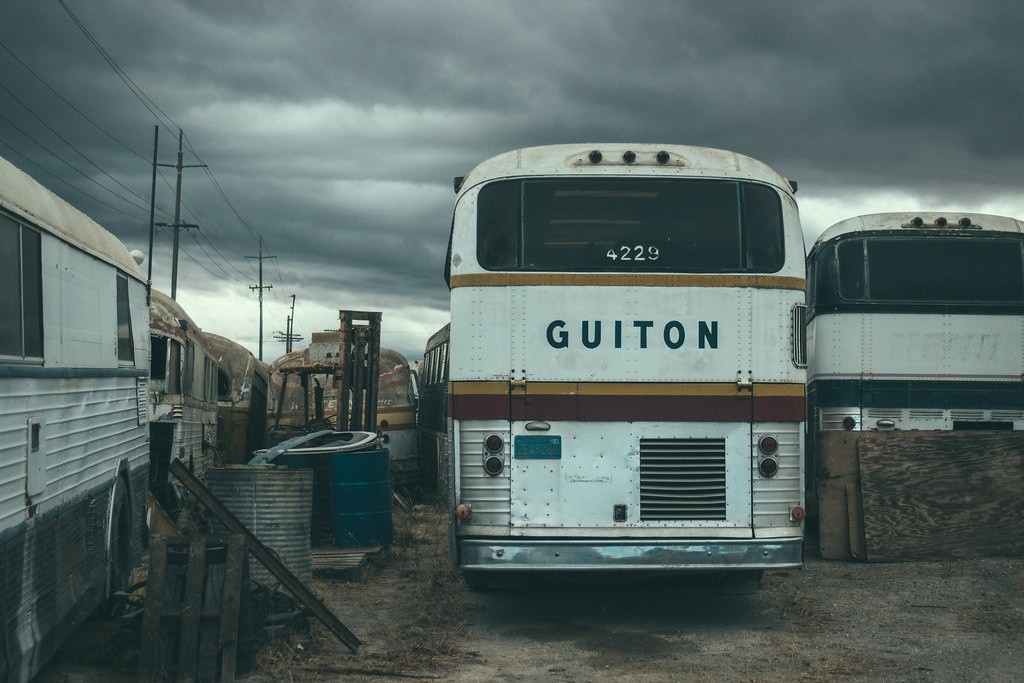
[207,468,314,608]
[329,448,394,549]
[269,454,322,547]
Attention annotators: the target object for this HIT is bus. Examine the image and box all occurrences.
[804,210,1024,431]
[416,141,810,592]
[0,155,416,681]
